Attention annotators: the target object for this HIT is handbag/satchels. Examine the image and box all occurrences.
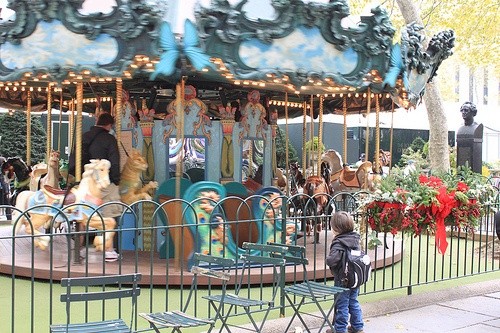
[68,130,103,179]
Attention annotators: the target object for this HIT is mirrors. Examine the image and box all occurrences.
[240,137,265,186]
[166,136,207,182]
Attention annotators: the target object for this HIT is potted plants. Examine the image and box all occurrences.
[304,136,325,159]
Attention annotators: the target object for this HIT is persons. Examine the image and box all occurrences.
[68,112,124,262]
[356,153,365,168]
[325,211,370,333]
[459,102,477,126]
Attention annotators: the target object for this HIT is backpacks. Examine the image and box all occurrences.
[330,244,372,289]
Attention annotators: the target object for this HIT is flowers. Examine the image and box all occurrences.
[363,170,489,255]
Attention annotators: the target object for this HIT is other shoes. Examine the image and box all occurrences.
[105,250,123,260]
[348,325,365,333]
[326,329,346,333]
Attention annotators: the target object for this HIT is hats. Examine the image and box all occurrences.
[0,157,5,165]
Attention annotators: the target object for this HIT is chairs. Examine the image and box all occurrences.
[49,241,350,333]
